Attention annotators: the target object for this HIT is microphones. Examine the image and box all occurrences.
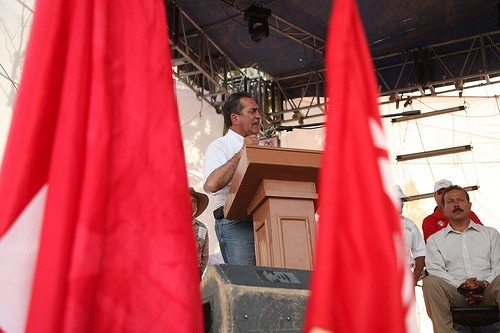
[265,125,277,138]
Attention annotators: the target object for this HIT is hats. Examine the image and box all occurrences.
[434,179,452,193]
[397,185,410,198]
[189,187,209,218]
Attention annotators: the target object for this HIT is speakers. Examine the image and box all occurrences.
[200,263,314,333]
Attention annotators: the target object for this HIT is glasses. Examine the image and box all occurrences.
[243,130,267,141]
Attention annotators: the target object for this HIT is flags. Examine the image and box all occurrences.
[304,0,422,333]
[1,0,206,333]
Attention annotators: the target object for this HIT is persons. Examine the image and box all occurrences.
[425,185,500,333]
[189,186,212,333]
[422,180,483,245]
[204,90,281,265]
[396,186,426,288]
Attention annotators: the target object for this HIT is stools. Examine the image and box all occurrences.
[453,306,500,327]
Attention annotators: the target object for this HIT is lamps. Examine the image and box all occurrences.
[243,6,273,43]
